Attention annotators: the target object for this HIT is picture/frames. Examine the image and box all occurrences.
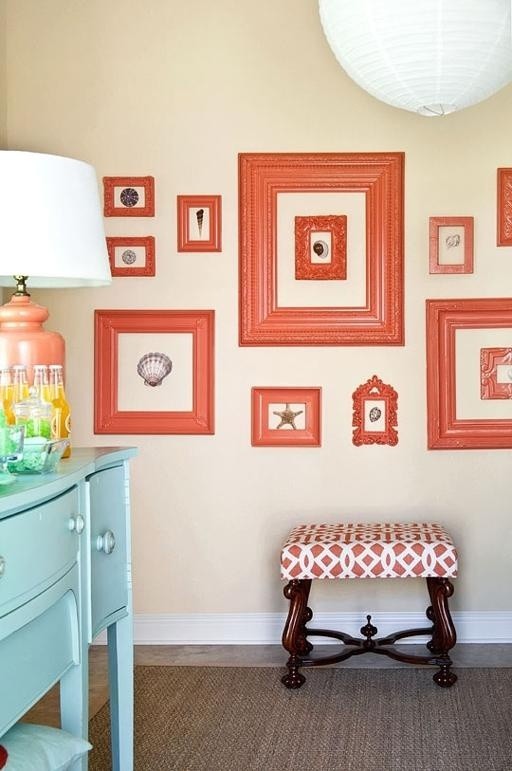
[106,236,156,277]
[430,216,474,273]
[176,194,222,252]
[252,386,320,447]
[104,177,154,217]
[238,152,406,347]
[353,375,398,447]
[424,297,512,451]
[94,310,216,435]
[496,168,512,248]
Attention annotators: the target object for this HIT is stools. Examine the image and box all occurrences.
[280,522,458,689]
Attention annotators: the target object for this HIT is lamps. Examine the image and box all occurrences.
[0,150,110,386]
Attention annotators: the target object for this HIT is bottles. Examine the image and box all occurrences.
[14,365,28,402]
[0,369,16,424]
[49,365,71,457]
[34,366,57,439]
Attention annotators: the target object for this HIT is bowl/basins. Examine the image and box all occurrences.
[1,432,69,476]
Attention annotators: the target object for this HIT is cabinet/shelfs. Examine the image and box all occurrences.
[0,447,140,771]
